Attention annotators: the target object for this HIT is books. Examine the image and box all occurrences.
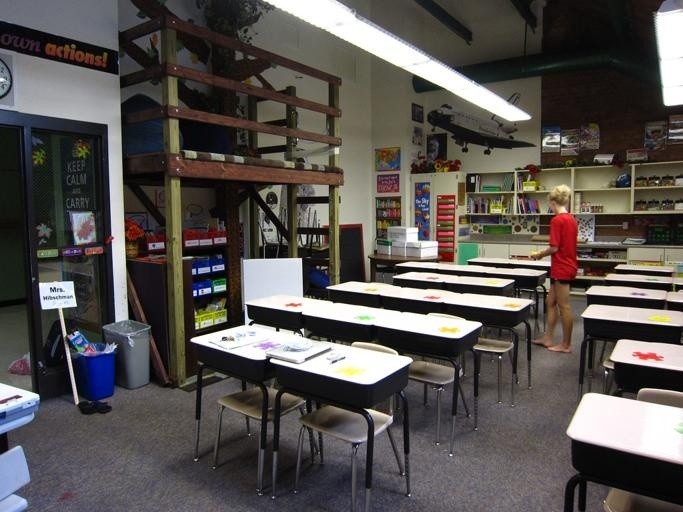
[467,173,551,213]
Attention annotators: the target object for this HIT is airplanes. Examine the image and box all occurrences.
[426,92,536,154]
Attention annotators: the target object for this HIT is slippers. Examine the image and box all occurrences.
[78,400,97,415]
[93,402,112,413]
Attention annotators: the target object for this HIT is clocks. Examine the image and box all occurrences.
[0,58,12,100]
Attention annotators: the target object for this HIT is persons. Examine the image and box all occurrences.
[428,139,440,161]
[531,184,579,352]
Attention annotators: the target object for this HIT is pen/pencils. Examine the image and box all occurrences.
[332,356,345,364]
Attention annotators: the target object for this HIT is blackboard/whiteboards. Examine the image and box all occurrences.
[322,224,366,283]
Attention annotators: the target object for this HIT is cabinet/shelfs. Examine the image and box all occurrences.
[409,161,682,297]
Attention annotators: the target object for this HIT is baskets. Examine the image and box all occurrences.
[192,258,225,275]
[193,278,226,297]
[194,308,227,329]
[146,232,227,250]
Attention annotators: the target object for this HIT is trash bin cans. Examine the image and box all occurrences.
[70,343,118,401]
[102,319,152,390]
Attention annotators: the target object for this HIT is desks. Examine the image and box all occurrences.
[563,264,682,512]
[367,253,442,282]
[189,258,551,512]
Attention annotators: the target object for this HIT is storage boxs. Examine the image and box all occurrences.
[376,226,439,257]
